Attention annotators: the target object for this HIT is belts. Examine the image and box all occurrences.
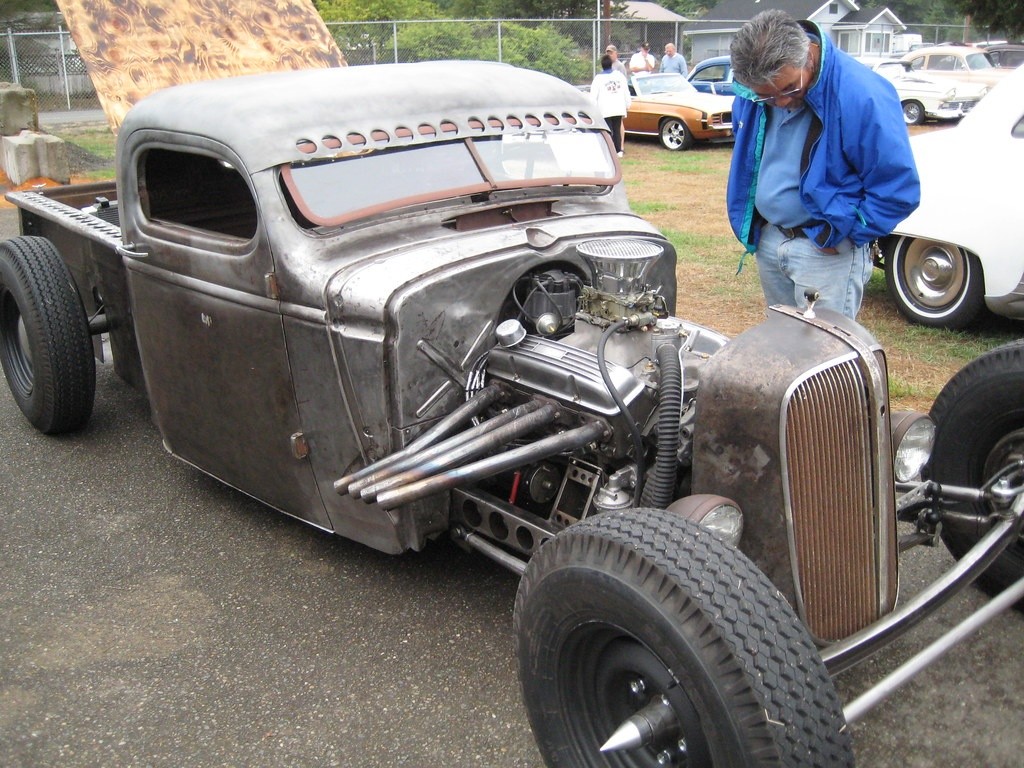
[776,225,807,239]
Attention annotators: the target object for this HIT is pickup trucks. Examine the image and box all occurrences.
[0,62,1024,768]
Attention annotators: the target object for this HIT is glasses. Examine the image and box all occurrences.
[750,68,803,103]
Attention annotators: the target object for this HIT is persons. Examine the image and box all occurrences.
[629,42,655,75]
[726,9,921,320]
[589,45,631,157]
[660,42,688,79]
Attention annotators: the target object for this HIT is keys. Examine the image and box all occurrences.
[869,242,883,263]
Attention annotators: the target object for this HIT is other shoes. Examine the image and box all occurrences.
[617,151,624,157]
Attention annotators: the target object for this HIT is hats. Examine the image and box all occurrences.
[641,42,650,50]
[606,45,617,52]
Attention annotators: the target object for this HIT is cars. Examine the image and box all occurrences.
[863,61,1024,326]
[868,42,1024,125]
[690,52,738,98]
[575,73,738,153]
